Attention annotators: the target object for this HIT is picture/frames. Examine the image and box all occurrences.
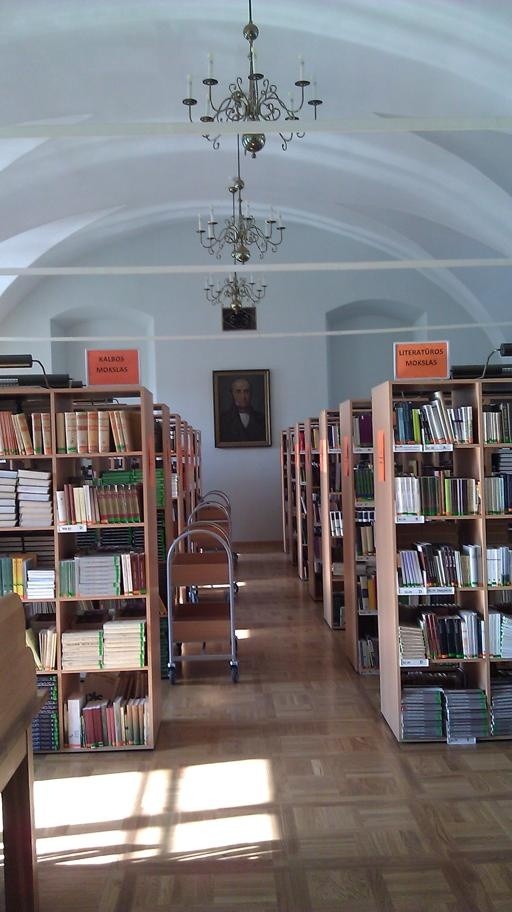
[212,368,272,448]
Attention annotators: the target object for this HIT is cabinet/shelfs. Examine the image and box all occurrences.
[0,378,202,911]
[281,378,512,744]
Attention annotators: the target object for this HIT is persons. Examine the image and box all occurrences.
[219,378,266,442]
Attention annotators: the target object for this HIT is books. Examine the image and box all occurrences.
[398,595,486,661]
[59,528,146,597]
[63,673,146,749]
[487,541,510,587]
[490,675,510,736]
[283,416,380,670]
[55,458,143,526]
[170,464,178,498]
[1,535,56,599]
[155,512,166,565]
[401,671,491,736]
[154,463,165,506]
[60,601,145,670]
[169,428,176,453]
[488,591,511,659]
[393,391,477,444]
[482,395,511,446]
[158,615,169,679]
[396,540,484,587]
[28,674,61,749]
[394,462,482,517]
[22,602,58,670]
[1,468,54,529]
[0,409,135,455]
[155,416,163,452]
[484,448,510,515]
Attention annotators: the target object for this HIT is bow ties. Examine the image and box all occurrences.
[240,408,249,412]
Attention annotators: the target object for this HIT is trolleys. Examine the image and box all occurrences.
[168,490,239,684]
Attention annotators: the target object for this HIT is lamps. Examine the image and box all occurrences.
[182,0,323,159]
[196,136,286,315]
